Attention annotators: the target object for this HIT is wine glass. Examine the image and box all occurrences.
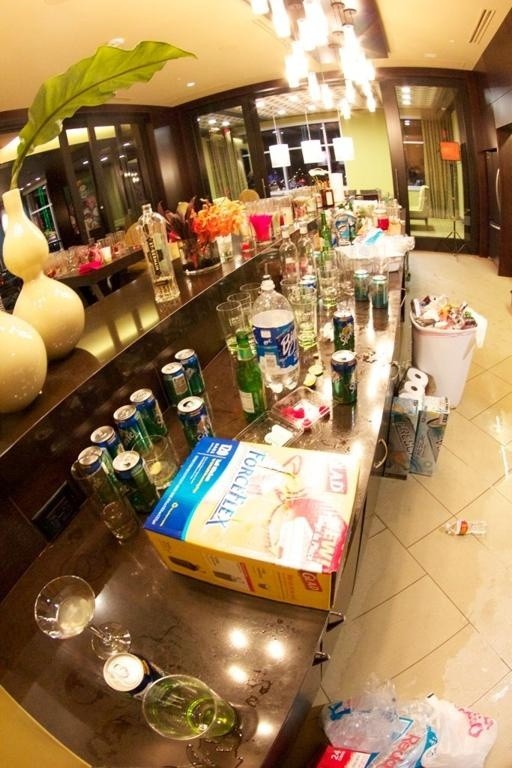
[33,574,130,658]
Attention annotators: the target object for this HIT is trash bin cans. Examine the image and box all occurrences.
[410,304,487,409]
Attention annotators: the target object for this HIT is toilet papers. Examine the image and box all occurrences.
[397,366,429,406]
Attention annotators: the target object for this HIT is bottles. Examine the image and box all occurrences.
[445,518,488,537]
[297,221,316,277]
[234,329,268,422]
[250,274,300,393]
[277,224,298,277]
[136,204,180,302]
[319,212,333,260]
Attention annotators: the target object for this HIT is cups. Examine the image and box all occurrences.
[70,453,139,541]
[46,230,127,277]
[376,191,401,230]
[130,434,178,492]
[212,696,258,746]
[216,232,234,261]
[237,183,334,241]
[216,250,338,355]
[340,250,390,296]
[117,530,171,599]
[140,672,234,742]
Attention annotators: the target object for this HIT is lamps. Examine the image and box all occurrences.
[264,103,357,170]
[438,140,468,257]
[238,0,392,120]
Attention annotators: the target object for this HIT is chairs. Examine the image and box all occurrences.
[407,183,433,232]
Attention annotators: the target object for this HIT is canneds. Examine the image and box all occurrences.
[353,269,370,301]
[102,652,164,700]
[333,310,355,352]
[348,221,356,238]
[298,275,317,288]
[371,275,389,309]
[312,251,321,269]
[77,348,215,513]
[331,350,358,405]
